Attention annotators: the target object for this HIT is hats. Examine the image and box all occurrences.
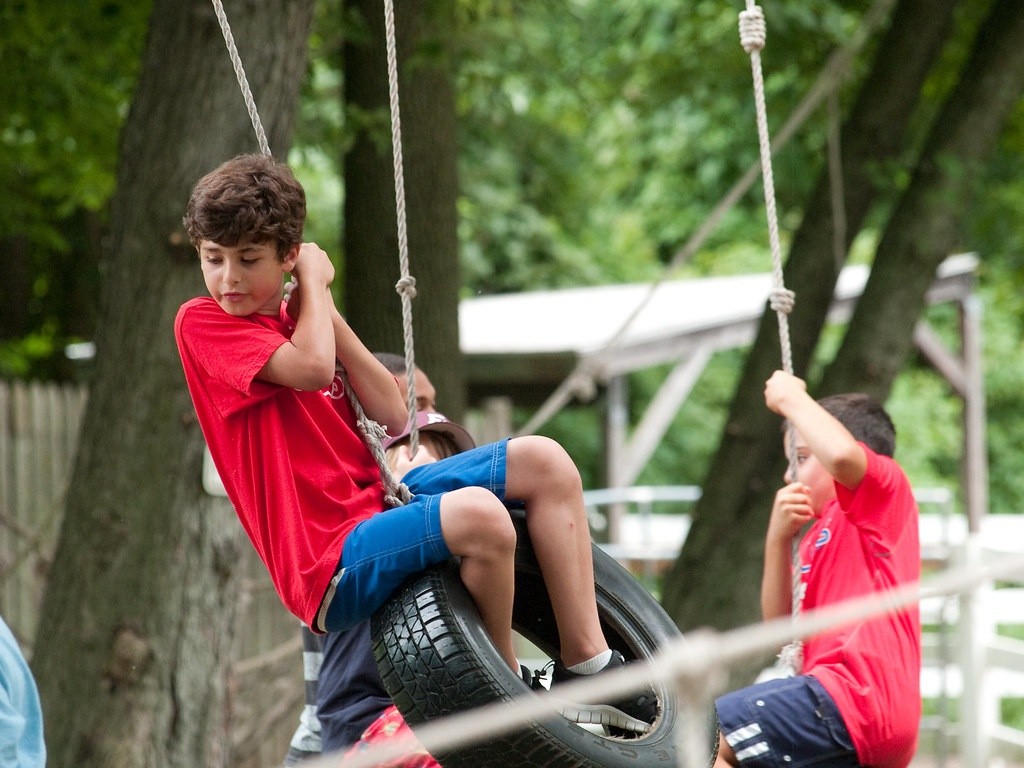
[381,412,475,453]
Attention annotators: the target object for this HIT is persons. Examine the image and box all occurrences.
[283,355,478,768]
[174,153,658,739]
[710,371,921,768]
[0,614,48,768]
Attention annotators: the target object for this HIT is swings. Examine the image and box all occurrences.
[209,2,720,768]
[739,0,857,767]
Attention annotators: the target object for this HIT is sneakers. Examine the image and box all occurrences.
[539,650,658,732]
[519,664,549,700]
